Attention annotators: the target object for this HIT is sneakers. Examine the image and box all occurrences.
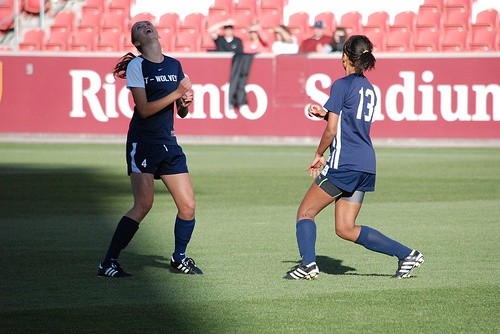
[96,259,132,279]
[170,253,204,275]
[391,250,425,279]
[282,262,319,281]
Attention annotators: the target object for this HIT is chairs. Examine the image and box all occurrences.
[0,0,500,54]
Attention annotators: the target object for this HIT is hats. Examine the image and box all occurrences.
[311,20,324,28]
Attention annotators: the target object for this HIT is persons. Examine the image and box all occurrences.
[282,34,425,280]
[97,20,204,278]
[205,17,348,56]
